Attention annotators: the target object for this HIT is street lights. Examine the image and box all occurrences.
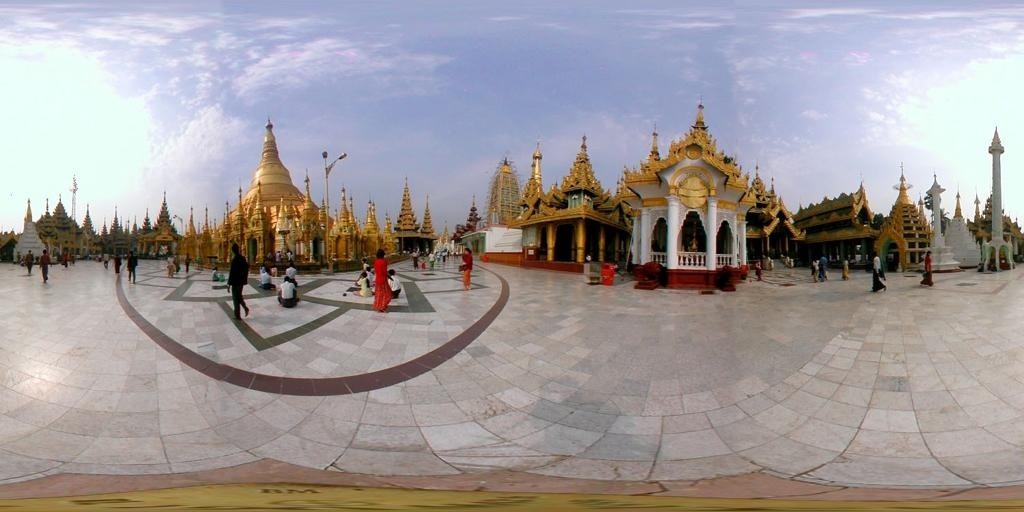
[172,215,184,235]
[321,147,351,273]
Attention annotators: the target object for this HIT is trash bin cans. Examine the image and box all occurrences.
[583,262,615,286]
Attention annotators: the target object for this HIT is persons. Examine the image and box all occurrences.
[812,251,934,291]
